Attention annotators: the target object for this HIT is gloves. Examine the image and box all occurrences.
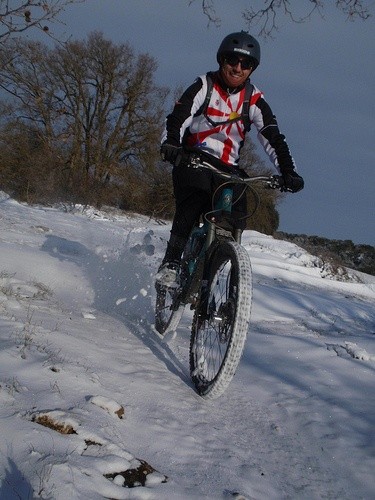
[281,169,305,193]
[160,141,181,164]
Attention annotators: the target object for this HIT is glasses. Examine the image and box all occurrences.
[220,54,256,69]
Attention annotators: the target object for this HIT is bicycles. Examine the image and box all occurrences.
[151,144,298,400]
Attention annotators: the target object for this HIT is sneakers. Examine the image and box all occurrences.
[156,258,181,289]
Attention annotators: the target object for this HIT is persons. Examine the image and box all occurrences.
[155,32,305,318]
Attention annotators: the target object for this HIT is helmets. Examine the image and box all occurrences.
[217,29,261,65]
